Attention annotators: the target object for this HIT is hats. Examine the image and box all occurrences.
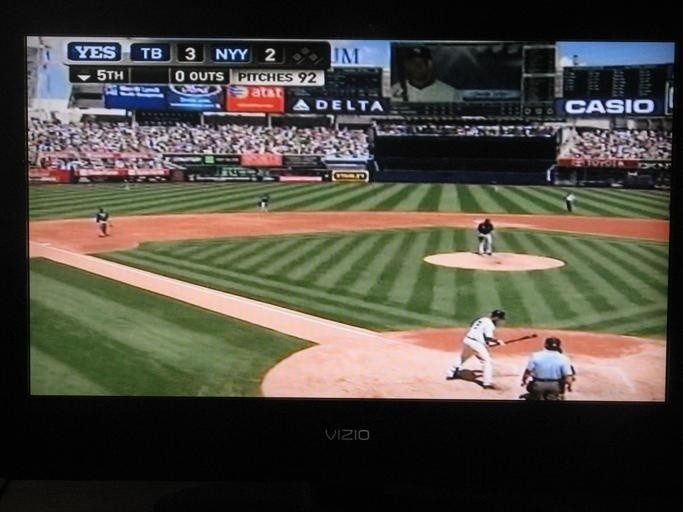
[546,338,559,350]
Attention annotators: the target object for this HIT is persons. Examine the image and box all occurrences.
[477,217,495,255]
[449,308,518,387]
[95,208,109,237]
[28,120,371,177]
[258,192,271,215]
[392,46,463,103]
[563,190,574,212]
[521,336,575,400]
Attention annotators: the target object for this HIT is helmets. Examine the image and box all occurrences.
[492,309,504,319]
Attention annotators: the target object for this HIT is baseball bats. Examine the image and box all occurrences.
[489,334,536,345]
